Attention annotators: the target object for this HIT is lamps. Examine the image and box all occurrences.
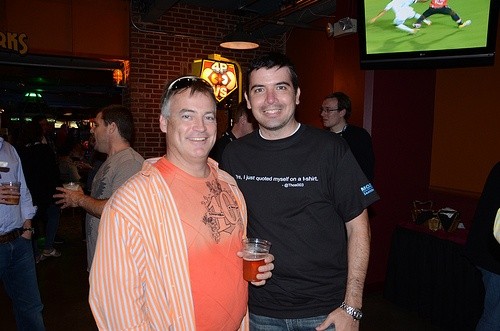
[218,26,259,51]
[326,17,357,38]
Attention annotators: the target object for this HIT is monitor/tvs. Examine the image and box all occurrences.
[356,0,500,69]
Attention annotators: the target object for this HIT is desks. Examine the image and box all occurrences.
[380,218,486,331]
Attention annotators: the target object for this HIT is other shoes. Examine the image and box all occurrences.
[457,19,471,29]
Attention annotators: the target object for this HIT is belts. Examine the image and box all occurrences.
[0,226,22,244]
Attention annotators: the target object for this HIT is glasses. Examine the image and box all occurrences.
[320,107,338,113]
[164,74,214,101]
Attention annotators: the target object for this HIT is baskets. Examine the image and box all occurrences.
[410,199,461,232]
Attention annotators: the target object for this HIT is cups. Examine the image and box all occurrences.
[2,182,21,205]
[241,238,271,283]
[63,182,83,207]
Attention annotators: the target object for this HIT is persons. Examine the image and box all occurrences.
[467,160,500,331]
[22,116,62,257]
[219,52,371,331]
[207,102,260,166]
[368,0,471,33]
[0,137,46,331]
[89,77,275,331]
[53,103,146,271]
[320,90,378,211]
[59,146,95,182]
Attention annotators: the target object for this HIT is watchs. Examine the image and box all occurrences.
[340,302,362,320]
